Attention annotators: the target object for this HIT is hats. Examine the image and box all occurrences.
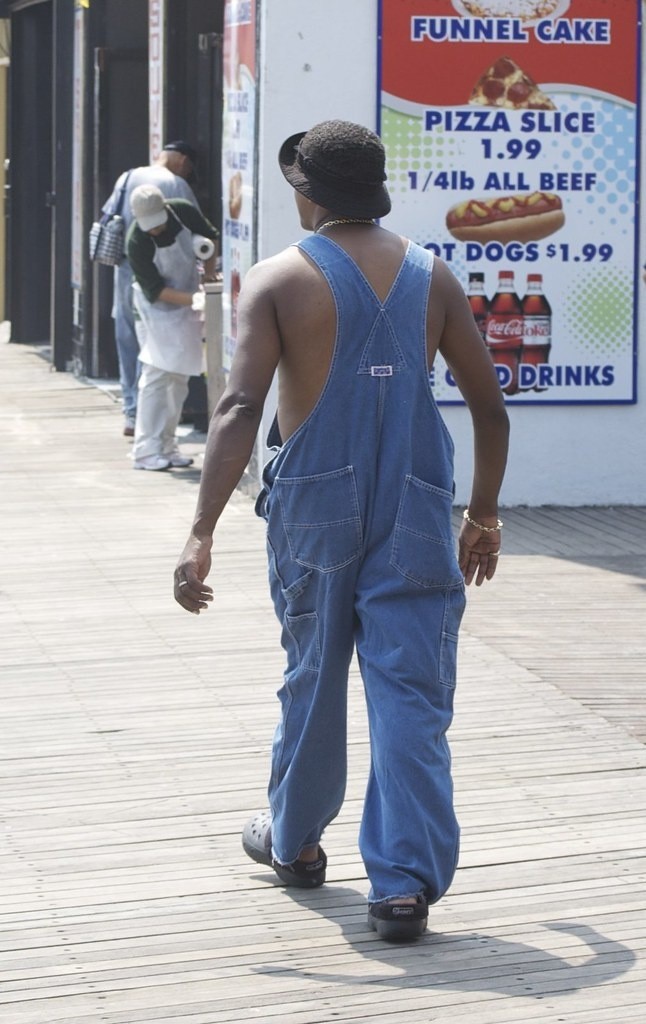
[278,118,396,219]
[162,138,197,165]
[130,183,168,233]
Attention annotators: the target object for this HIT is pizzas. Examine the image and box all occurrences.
[468,55,557,110]
[461,0,558,22]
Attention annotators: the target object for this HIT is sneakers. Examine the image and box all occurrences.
[164,452,194,467]
[133,453,170,470]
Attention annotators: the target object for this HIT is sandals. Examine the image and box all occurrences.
[367,894,431,946]
[241,809,330,888]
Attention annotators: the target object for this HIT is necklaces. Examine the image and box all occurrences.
[316,218,378,233]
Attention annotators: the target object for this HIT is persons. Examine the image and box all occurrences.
[125,186,221,469]
[103,134,199,438]
[174,121,511,944]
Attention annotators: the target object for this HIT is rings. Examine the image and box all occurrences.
[178,581,187,587]
[488,552,500,555]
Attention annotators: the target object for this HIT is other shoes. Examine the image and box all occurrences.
[124,427,135,434]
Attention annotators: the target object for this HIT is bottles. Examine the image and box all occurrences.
[522,274,552,392]
[230,248,241,338]
[485,271,525,395]
[467,272,490,340]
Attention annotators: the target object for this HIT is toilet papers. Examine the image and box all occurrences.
[193,235,215,260]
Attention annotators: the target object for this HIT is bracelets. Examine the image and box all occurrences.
[463,507,504,532]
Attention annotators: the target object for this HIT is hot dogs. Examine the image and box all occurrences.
[446,191,564,244]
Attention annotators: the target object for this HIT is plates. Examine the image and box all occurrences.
[451,0,571,29]
